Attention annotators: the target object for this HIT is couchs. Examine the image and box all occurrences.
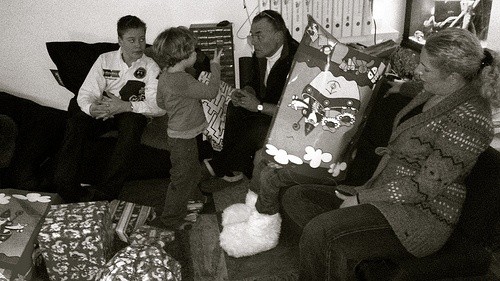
[0,33,500,281]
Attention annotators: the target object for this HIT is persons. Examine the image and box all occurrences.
[76,14,167,200]
[281,28,500,281]
[153,26,223,230]
[219,149,347,258]
[205,10,300,182]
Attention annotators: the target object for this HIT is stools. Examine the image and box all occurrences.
[38,202,114,281]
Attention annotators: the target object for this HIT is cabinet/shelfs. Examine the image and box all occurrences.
[189,23,235,88]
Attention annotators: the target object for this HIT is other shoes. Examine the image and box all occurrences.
[62,183,90,203]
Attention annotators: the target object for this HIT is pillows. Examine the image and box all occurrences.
[261,13,392,181]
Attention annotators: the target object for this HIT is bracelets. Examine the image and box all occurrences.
[356,193,360,204]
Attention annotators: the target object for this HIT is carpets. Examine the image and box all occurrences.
[110,200,157,242]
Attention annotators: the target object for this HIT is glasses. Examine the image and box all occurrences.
[256,11,279,23]
